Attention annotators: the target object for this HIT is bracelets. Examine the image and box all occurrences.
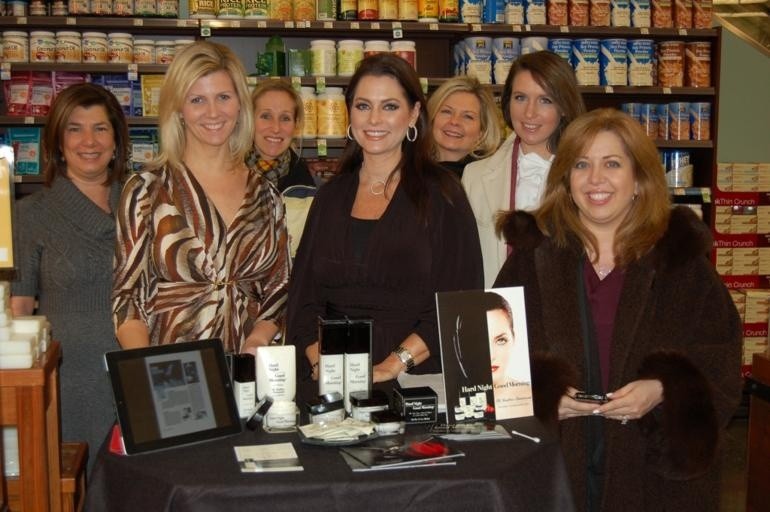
[392,345,416,373]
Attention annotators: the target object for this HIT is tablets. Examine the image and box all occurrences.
[104,338,245,458]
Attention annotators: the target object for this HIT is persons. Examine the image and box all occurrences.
[7,83,134,512]
[444,291,534,423]
[109,41,294,393]
[239,78,318,198]
[459,49,590,292]
[492,106,744,512]
[424,76,499,183]
[285,50,483,424]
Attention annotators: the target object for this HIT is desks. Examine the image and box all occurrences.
[78,397,581,510]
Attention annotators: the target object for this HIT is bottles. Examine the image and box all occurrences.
[0,30,197,70]
[263,34,419,79]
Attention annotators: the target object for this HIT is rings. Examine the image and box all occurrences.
[620,414,630,425]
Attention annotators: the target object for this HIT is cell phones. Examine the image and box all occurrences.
[575,392,609,405]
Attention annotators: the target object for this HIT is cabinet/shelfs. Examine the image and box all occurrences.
[1,328,91,511]
[0,7,723,253]
[709,188,770,383]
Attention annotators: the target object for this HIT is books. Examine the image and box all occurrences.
[230,432,466,474]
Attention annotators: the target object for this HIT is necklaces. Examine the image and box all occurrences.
[520,144,547,161]
[361,156,401,196]
[588,248,618,278]
